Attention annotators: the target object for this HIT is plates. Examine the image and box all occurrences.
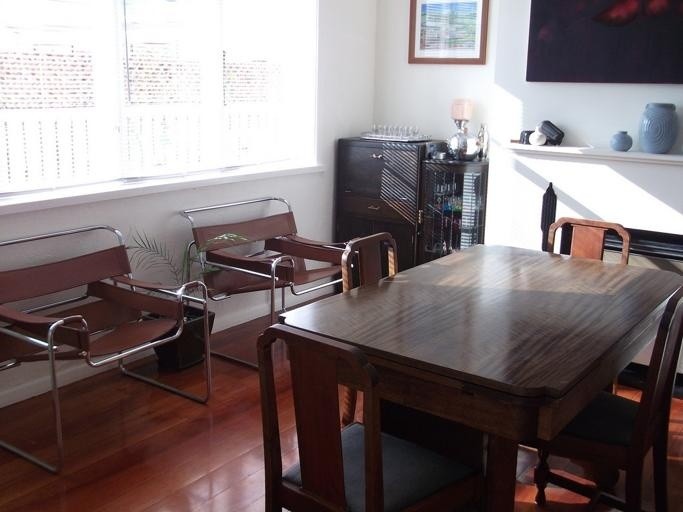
[360,133,431,142]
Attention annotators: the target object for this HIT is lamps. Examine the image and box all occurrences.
[447,97,476,164]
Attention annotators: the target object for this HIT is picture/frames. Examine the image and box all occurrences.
[409,2,487,66]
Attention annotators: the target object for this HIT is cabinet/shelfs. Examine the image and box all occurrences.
[340,135,448,286]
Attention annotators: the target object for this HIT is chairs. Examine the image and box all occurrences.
[536,286,683,506]
[2,223,215,477]
[538,219,635,265]
[177,192,358,369]
[251,322,494,507]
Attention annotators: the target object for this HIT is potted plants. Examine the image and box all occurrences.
[122,228,250,376]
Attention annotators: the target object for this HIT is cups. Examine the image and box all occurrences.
[373,125,421,138]
